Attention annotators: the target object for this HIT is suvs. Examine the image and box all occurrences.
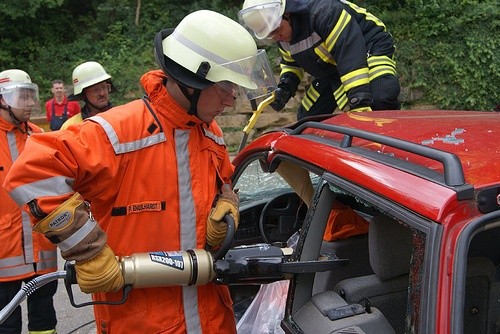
[225,109,500,334]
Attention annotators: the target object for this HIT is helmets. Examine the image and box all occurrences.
[0,69,33,95]
[72,60,110,96]
[153,9,258,90]
[242,0,286,40]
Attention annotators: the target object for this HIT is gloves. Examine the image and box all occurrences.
[207,189,239,246]
[348,92,373,111]
[32,192,124,294]
[268,78,296,112]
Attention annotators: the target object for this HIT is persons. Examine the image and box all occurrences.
[59,61,114,131]
[45,79,82,131]
[0,68,59,334]
[2,9,280,334]
[239,0,416,223]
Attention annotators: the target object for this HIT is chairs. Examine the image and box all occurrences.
[336,215,495,334]
